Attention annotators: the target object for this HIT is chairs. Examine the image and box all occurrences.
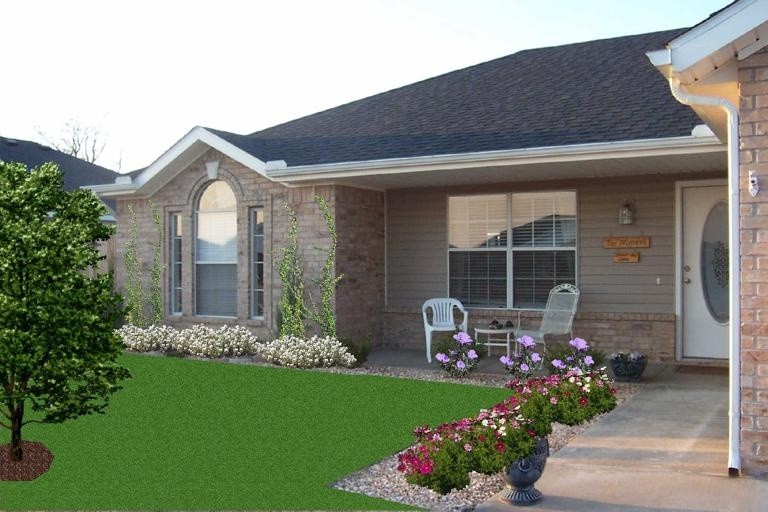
[515,284,580,367]
[422,298,468,363]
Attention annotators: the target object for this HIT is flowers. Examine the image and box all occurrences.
[611,351,644,360]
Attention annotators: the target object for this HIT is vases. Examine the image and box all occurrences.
[609,359,648,382]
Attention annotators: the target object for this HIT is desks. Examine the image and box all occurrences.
[474,327,517,359]
